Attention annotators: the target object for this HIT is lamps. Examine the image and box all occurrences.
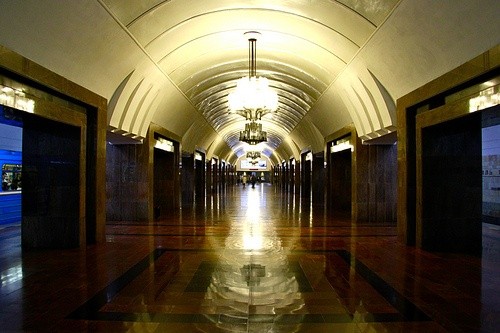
[228,30,279,165]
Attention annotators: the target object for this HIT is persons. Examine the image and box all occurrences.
[0,172,22,192]
